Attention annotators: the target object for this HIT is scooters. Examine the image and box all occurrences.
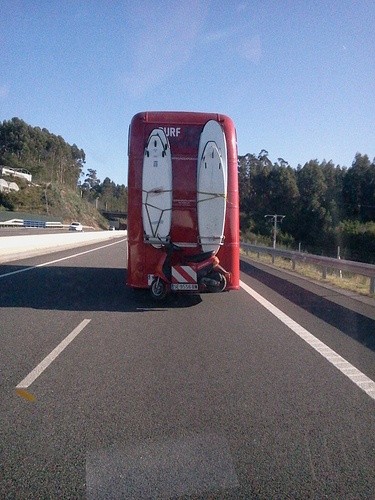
[152,233,230,299]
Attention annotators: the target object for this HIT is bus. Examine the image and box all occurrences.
[127,110,242,294]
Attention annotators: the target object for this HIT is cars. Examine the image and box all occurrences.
[69,222,83,232]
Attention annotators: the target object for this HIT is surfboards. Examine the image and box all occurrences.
[141,127,172,248]
[197,119,228,256]
